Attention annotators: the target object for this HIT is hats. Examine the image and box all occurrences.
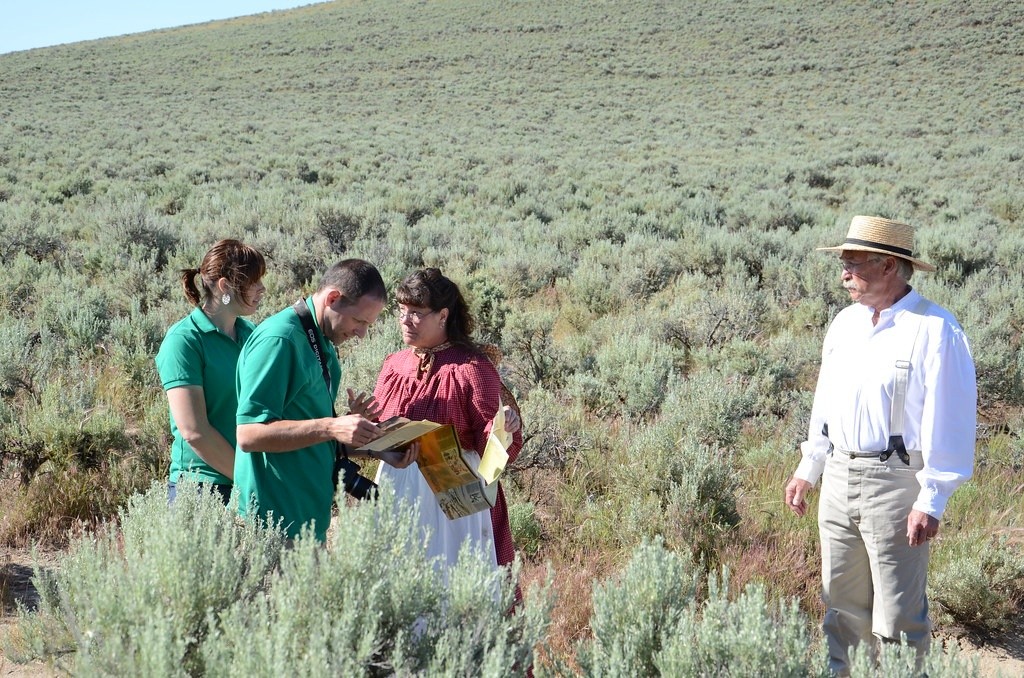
[816,216,938,273]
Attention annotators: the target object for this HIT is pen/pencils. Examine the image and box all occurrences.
[375,416,397,429]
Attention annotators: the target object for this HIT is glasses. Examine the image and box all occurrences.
[836,258,876,274]
[392,308,434,321]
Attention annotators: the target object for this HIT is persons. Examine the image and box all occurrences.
[347,268,534,678]
[226,258,423,548]
[154,239,266,505]
[785,216,976,678]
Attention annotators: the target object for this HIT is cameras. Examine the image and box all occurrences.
[332,458,379,508]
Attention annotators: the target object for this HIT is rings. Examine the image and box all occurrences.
[927,537,932,540]
[364,410,371,415]
